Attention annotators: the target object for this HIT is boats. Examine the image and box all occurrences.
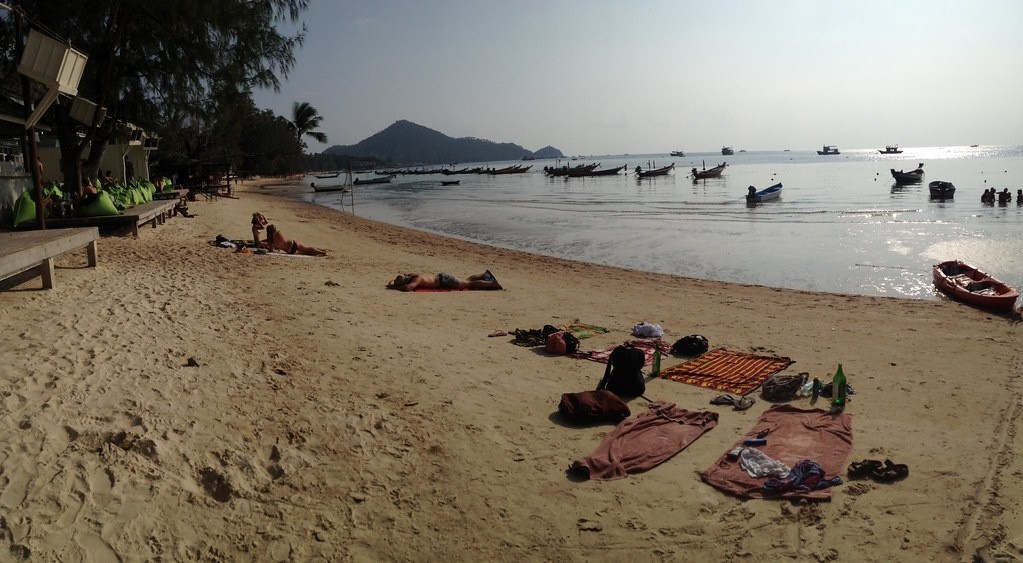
[722,147,735,156]
[521,155,535,162]
[878,145,904,154]
[931,259,1020,311]
[816,146,841,156]
[739,149,746,153]
[316,171,340,179]
[314,183,345,193]
[440,179,462,185]
[692,161,730,180]
[890,162,926,185]
[375,164,534,175]
[744,182,784,203]
[541,161,628,179]
[354,174,398,185]
[670,150,684,158]
[928,180,957,199]
[635,163,675,177]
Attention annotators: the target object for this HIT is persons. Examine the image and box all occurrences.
[251,212,268,245]
[266,224,326,255]
[981,187,1023,206]
[37,157,44,180]
[125,155,134,186]
[102,171,119,190]
[152,175,164,192]
[78,178,97,206]
[388,269,503,292]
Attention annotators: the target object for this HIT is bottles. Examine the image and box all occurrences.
[652,342,661,375]
[832,364,846,404]
[812,376,819,395]
